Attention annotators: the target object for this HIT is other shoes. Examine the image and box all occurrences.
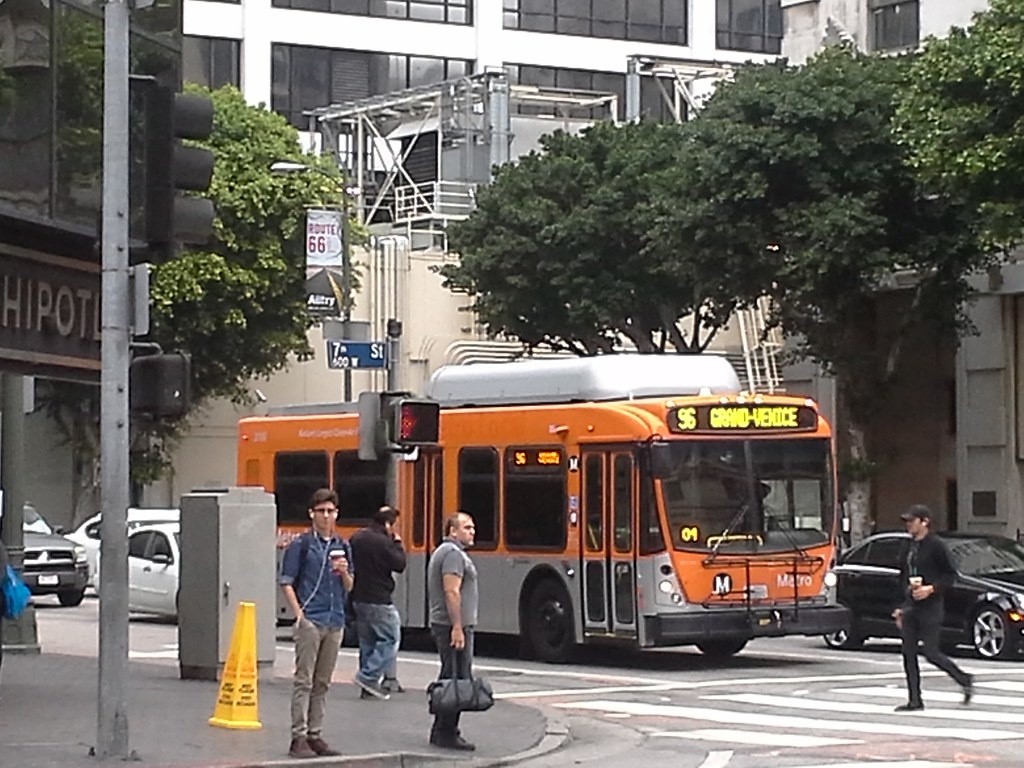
[351,672,390,700]
[289,738,317,758]
[306,738,341,755]
[894,700,924,711]
[430,734,475,751]
[963,674,972,702]
[360,688,377,699]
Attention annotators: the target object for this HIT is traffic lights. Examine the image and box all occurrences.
[144,84,217,266]
[392,398,441,447]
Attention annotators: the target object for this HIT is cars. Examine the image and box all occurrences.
[92,524,181,623]
[823,530,1024,660]
[23,503,90,609]
[63,508,181,587]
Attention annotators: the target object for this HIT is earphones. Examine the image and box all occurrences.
[334,513,337,519]
[311,513,314,518]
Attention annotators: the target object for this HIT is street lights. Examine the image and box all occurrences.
[269,161,353,401]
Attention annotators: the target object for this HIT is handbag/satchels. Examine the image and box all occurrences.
[425,646,494,713]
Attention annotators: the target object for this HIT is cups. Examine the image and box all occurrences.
[910,577,922,589]
[330,550,345,575]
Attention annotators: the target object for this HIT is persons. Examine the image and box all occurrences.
[278,489,354,758]
[893,505,973,711]
[428,512,479,750]
[349,506,406,699]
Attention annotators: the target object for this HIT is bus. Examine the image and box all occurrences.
[237,357,850,663]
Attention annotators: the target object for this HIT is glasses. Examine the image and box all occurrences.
[314,509,335,513]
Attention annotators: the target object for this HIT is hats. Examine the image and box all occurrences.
[900,504,933,522]
[374,505,400,525]
